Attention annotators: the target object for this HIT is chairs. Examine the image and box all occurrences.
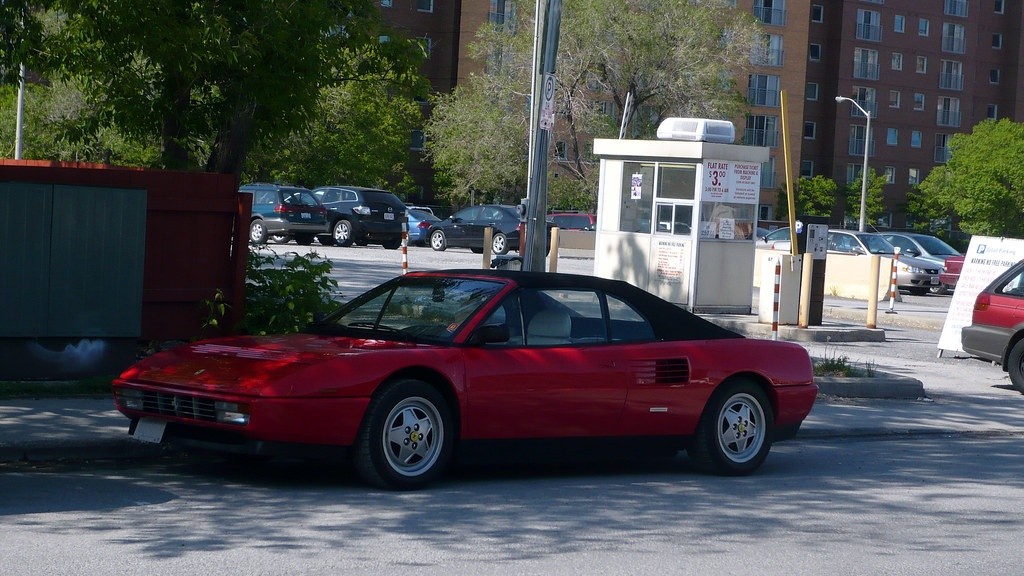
[526,310,574,346]
[409,297,457,342]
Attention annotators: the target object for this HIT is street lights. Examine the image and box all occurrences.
[836,96,871,233]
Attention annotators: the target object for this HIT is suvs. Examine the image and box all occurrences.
[239,182,331,246]
[269,186,410,250]
[960,259,1024,394]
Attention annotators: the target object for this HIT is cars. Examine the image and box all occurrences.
[402,208,443,247]
[111,267,820,497]
[772,229,942,296]
[756,227,797,250]
[425,205,557,256]
[542,214,596,233]
[642,221,691,233]
[871,232,965,294]
